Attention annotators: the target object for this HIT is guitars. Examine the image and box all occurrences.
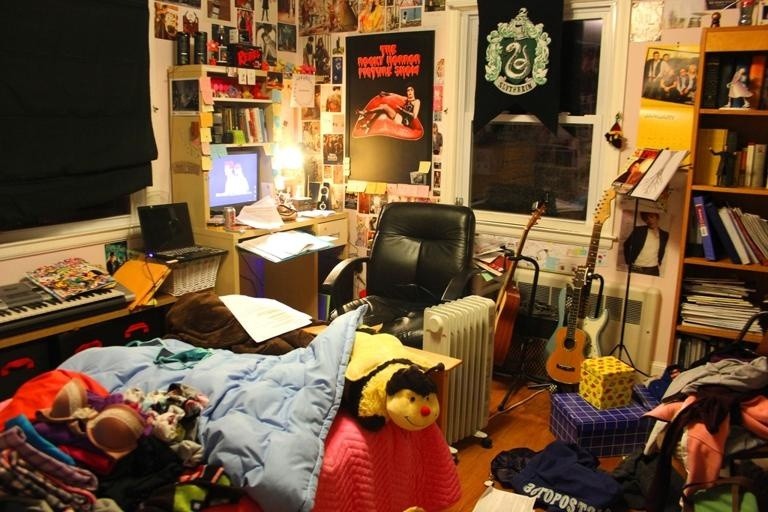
[495,204,545,366]
[545,266,591,385]
[543,187,616,358]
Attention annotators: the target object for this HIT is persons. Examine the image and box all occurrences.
[612,158,642,186]
[260,33,277,66]
[358,86,420,132]
[623,212,670,280]
[641,50,698,103]
[325,86,341,112]
[106,252,126,274]
[432,122,444,155]
[301,36,313,68]
[238,9,252,42]
[313,37,331,76]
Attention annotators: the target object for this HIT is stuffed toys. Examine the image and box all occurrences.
[337,326,442,434]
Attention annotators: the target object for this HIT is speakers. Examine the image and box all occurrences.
[310,181,332,211]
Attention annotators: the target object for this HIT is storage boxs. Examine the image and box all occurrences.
[638,374,664,416]
[549,391,649,458]
[579,356,636,411]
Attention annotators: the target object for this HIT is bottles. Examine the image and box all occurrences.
[224,207,236,227]
[177,31,190,65]
[738,0,755,26]
[195,32,207,64]
[217,24,227,63]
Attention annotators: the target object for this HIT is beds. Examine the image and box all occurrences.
[0,321,463,512]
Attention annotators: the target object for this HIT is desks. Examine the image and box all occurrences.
[0,289,215,351]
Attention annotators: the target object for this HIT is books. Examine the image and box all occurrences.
[235,230,335,265]
[298,208,336,220]
[696,125,767,189]
[211,106,271,144]
[111,258,173,313]
[26,257,116,303]
[693,194,768,267]
[680,275,768,336]
[612,147,691,202]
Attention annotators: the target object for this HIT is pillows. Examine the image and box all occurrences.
[57,303,368,511]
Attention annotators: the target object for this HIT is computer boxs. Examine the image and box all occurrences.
[320,259,355,315]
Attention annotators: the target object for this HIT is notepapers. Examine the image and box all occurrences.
[346,180,429,198]
[216,145,228,158]
[210,145,219,160]
[200,111,213,127]
[271,89,281,104]
[200,76,212,91]
[200,127,212,143]
[262,142,273,156]
[202,102,215,112]
[237,68,247,86]
[418,161,431,173]
[201,142,211,156]
[248,68,256,86]
[227,67,238,77]
[201,89,214,104]
[201,156,211,172]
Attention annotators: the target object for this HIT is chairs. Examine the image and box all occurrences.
[321,199,477,345]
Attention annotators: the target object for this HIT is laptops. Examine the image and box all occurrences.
[138,201,229,268]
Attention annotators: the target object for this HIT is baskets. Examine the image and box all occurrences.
[127,248,221,297]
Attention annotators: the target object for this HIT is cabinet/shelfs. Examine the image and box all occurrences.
[167,65,349,306]
[665,25,768,371]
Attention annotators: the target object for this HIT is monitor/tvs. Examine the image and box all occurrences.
[210,146,263,216]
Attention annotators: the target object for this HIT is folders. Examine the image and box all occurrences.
[704,202,741,263]
[112,259,169,311]
[688,205,696,257]
[696,223,704,257]
[137,268,173,307]
[718,206,751,265]
[692,196,728,261]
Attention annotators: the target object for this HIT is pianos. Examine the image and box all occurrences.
[0,280,137,335]
